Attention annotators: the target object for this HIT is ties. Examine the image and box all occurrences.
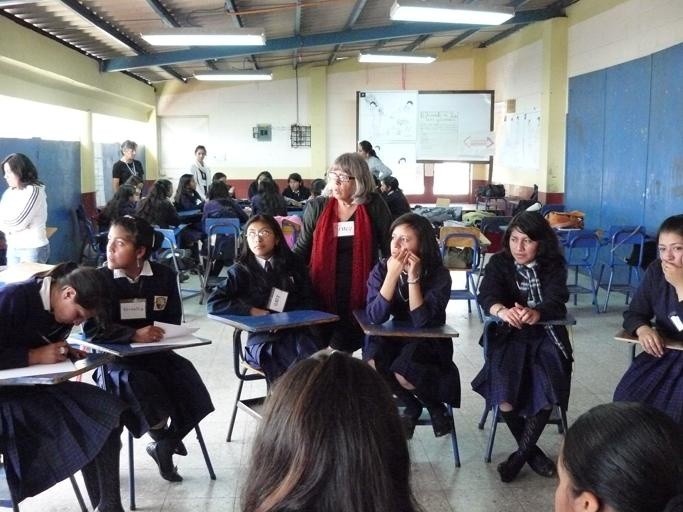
[264,260,272,277]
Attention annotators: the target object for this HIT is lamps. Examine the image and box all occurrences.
[138,26,267,47]
[386,0,516,26]
[191,68,273,82]
[357,49,438,67]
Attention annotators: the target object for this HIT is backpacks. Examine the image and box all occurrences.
[543,208,587,230]
[413,205,496,228]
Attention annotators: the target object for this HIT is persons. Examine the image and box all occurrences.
[289,151,393,357]
[612,213,683,423]
[237,347,426,512]
[77,214,215,483]
[471,210,574,483]
[1,260,132,512]
[0,151,51,267]
[553,400,683,512]
[364,212,462,440]
[204,212,327,390]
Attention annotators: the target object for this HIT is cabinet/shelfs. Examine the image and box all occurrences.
[475,197,510,217]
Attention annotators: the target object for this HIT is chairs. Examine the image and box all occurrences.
[69,323,217,510]
[592,225,657,313]
[0,354,116,511]
[198,218,241,305]
[352,309,460,467]
[478,217,511,236]
[76,205,109,265]
[478,314,576,464]
[437,198,450,208]
[150,224,201,302]
[540,204,565,217]
[614,326,682,366]
[436,227,490,324]
[206,309,340,442]
[553,227,611,314]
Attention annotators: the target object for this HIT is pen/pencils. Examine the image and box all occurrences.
[38,332,53,345]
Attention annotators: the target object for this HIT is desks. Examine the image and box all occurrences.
[410,203,477,211]
[288,211,304,218]
[47,227,58,240]
[177,209,200,216]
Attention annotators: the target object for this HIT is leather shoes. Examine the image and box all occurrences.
[402,403,423,439]
[526,445,555,478]
[498,448,527,482]
[147,440,184,482]
[426,402,453,438]
[147,424,188,456]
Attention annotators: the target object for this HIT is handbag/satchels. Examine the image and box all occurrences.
[441,245,476,269]
[476,183,507,197]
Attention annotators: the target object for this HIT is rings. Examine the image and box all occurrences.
[153,336,156,340]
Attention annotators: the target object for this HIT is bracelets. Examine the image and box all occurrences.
[406,277,421,284]
[496,306,506,318]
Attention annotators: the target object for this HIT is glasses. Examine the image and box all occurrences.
[244,230,274,239]
[327,171,355,182]
